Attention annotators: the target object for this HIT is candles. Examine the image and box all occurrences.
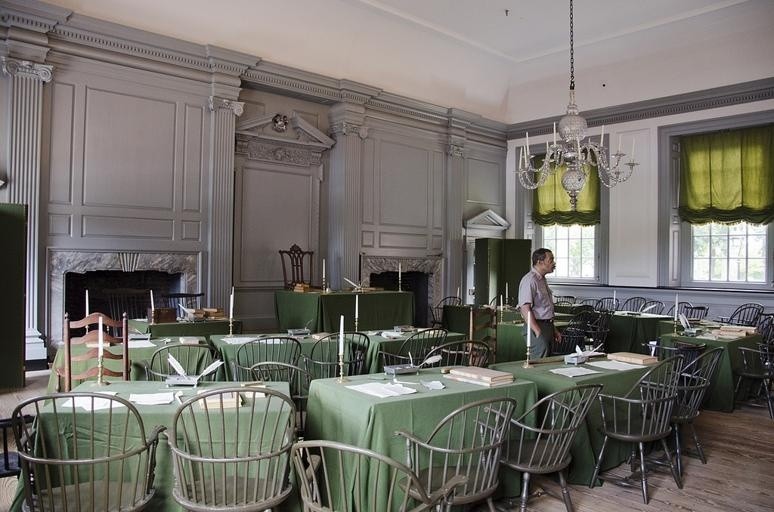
[323,258,325,278]
[399,263,401,280]
[506,283,508,298]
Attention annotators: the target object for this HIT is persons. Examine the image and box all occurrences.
[518,249,562,358]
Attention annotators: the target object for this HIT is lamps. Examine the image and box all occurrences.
[518,0,638,207]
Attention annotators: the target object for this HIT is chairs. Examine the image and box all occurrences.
[278,244,321,289]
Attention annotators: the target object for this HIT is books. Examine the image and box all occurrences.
[186,307,224,321]
[684,319,758,338]
[607,352,658,364]
[442,366,514,387]
[294,284,309,293]
